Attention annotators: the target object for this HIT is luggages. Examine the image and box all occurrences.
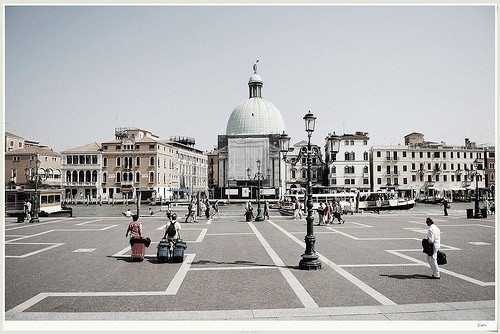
[173,238,184,262]
[157,238,169,261]
[131,237,145,260]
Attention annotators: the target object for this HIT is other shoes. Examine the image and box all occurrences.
[317,220,346,226]
[433,277,440,279]
[432,275,434,276]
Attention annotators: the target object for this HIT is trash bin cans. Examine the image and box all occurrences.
[466,208,473,218]
[480,208,487,218]
[18,212,24,222]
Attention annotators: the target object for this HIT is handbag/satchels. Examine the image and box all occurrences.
[437,250,447,264]
[130,237,151,248]
[159,241,171,247]
[175,240,187,250]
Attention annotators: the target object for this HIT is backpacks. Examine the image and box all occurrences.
[168,221,177,237]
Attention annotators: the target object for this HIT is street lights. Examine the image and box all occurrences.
[24,159,50,223]
[276,109,341,272]
[246,160,271,222]
[465,159,490,219]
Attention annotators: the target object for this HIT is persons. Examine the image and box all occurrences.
[184,197,221,223]
[164,209,174,220]
[292,199,303,220]
[243,200,255,218]
[423,217,441,279]
[125,215,145,241]
[149,208,154,215]
[122,209,132,217]
[163,213,182,262]
[263,199,269,221]
[440,197,448,216]
[485,199,495,215]
[317,198,346,225]
[24,200,32,221]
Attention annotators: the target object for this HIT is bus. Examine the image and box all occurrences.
[281,192,415,215]
